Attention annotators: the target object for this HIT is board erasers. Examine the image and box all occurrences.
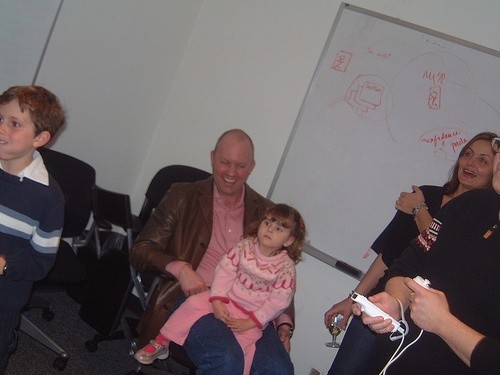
[335,260,362,278]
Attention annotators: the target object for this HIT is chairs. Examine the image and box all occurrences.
[19,147,97,365]
[87,165,212,375]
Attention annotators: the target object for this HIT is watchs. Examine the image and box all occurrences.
[411,202,428,216]
[277,324,293,338]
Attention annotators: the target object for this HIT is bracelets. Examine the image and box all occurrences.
[3,263,8,275]
[349,290,354,297]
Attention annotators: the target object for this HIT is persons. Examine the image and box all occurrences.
[0,84,70,373]
[351,137,500,375]
[324,132,499,374]
[129,128,296,375]
[134,202,306,374]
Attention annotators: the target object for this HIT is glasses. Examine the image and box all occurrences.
[491,137,499,152]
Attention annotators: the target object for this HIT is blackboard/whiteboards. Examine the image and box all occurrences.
[267,1,500,280]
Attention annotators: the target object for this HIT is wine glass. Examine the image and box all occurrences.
[326,311,343,349]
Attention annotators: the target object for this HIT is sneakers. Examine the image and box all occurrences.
[134,340,169,365]
